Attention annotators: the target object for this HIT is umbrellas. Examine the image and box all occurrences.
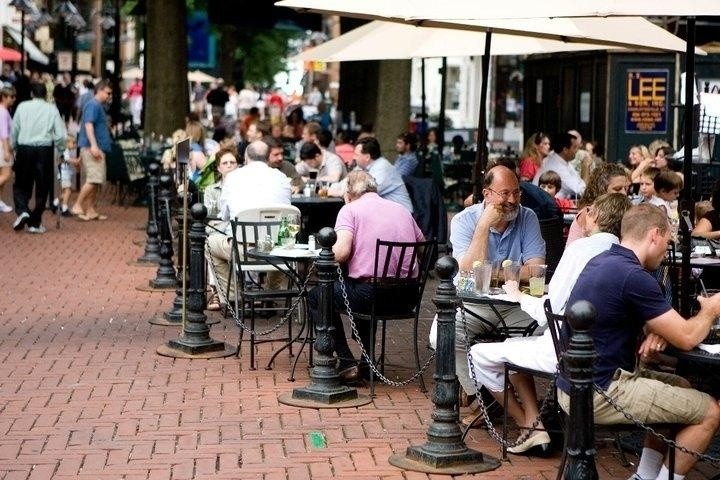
[291,20,622,154]
[273,0,709,206]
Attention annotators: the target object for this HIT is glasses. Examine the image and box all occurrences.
[488,185,522,200]
[103,89,113,95]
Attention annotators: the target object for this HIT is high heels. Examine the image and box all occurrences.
[508,431,552,456]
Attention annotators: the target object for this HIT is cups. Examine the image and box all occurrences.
[502,261,523,287]
[475,258,495,294]
[699,289,720,330]
[526,264,548,296]
[318,180,330,200]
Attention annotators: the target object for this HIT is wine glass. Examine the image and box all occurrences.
[286,214,301,248]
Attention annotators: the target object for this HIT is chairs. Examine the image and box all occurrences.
[231,220,311,371]
[334,234,435,398]
[111,142,147,209]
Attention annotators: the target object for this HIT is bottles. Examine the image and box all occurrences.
[277,216,289,247]
[303,183,310,198]
[309,234,316,254]
[310,184,315,198]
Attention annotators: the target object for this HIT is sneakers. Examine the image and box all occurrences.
[28,224,47,233]
[363,355,382,379]
[51,201,59,212]
[337,362,362,385]
[0,199,14,214]
[461,401,502,428]
[61,207,72,217]
[11,212,30,231]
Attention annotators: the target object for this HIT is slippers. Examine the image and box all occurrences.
[71,208,91,222]
[87,211,108,220]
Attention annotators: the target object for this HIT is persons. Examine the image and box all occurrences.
[1,68,144,234]
[157,68,720,479]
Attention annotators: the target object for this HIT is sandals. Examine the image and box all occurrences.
[208,294,221,311]
